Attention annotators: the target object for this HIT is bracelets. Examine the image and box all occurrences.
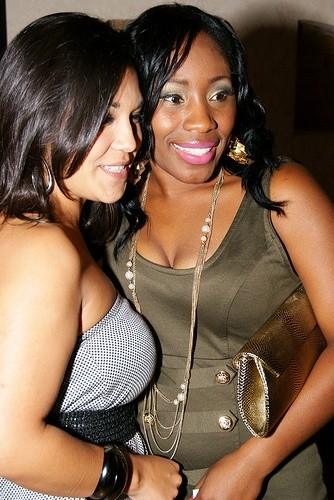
[85,443,116,500]
[104,443,128,500]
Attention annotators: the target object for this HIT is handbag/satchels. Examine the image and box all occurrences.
[231,284,327,438]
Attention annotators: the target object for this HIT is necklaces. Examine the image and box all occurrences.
[125,167,224,458]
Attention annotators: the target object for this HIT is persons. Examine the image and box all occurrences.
[100,3,334,500]
[0,11,182,500]
